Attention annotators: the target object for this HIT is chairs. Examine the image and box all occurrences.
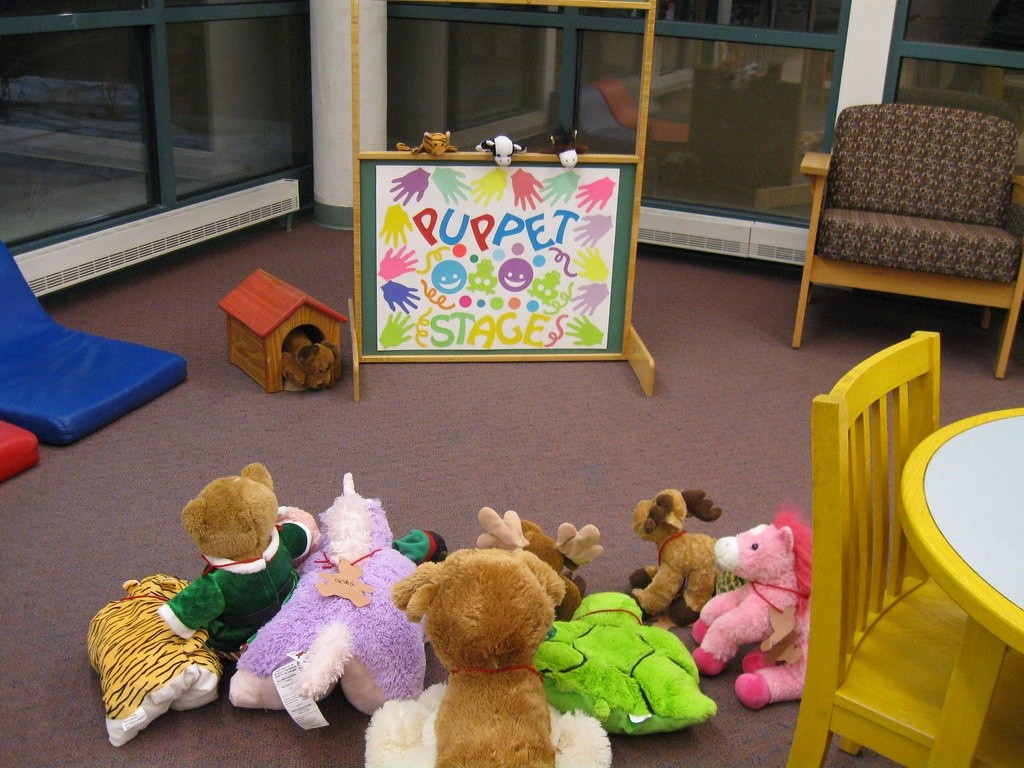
[790,103,1024,380]
[786,327,1024,768]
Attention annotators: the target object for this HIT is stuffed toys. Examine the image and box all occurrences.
[397,131,459,155]
[692,513,813,709]
[477,507,604,622]
[87,572,223,747]
[281,332,338,389]
[551,130,588,168]
[392,549,566,768]
[475,135,527,167]
[391,529,448,566]
[534,592,717,734]
[629,488,722,627]
[716,569,748,595]
[158,463,311,660]
[276,506,322,569]
[365,683,613,768]
[229,472,426,716]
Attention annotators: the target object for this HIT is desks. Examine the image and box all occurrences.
[898,407,1024,768]
